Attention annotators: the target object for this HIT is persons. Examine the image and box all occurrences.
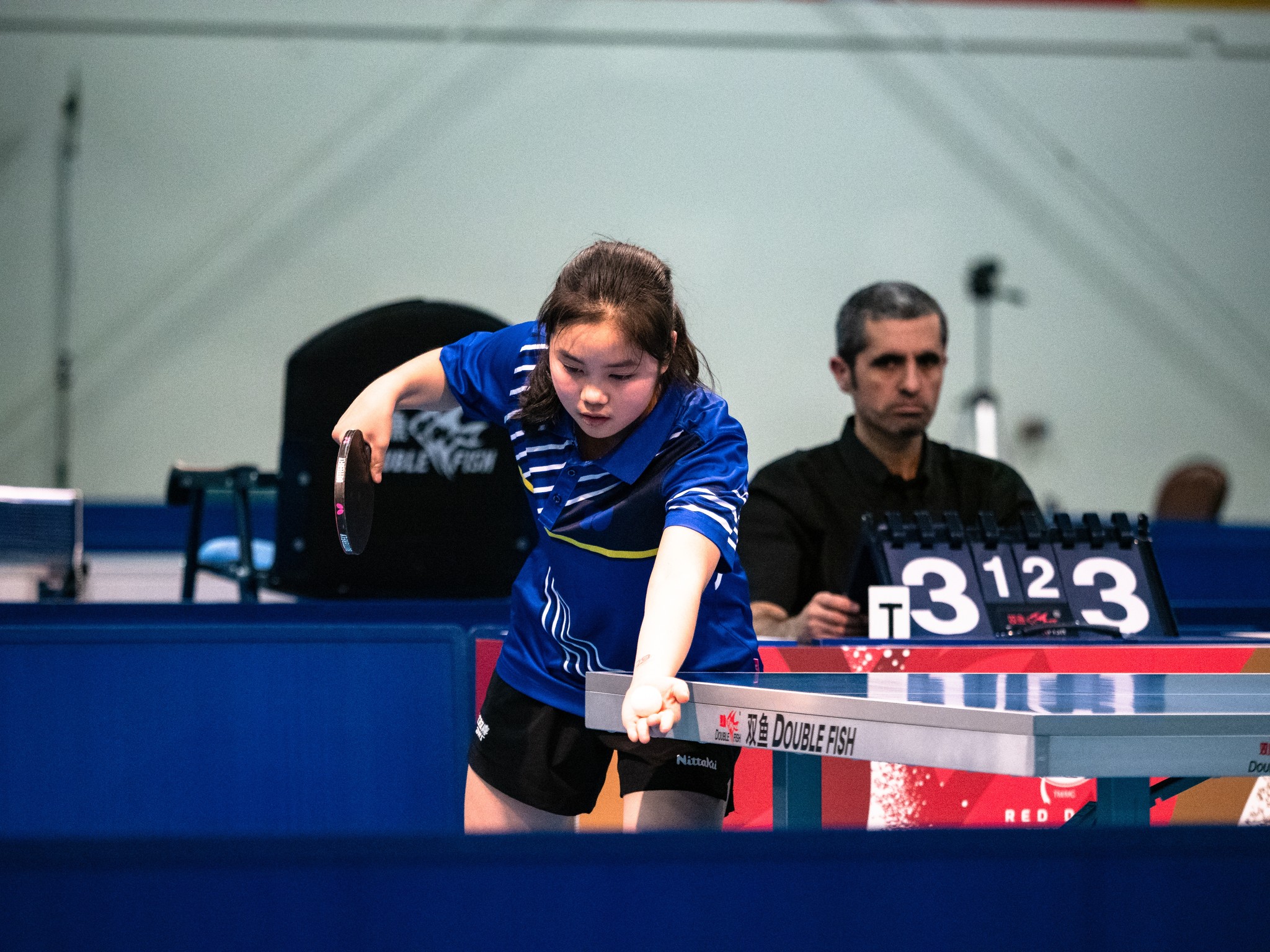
[736,282,1050,643]
[332,242,765,833]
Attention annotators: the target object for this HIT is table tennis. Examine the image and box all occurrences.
[631,684,666,720]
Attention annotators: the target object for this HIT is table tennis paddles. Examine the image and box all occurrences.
[332,425,375,556]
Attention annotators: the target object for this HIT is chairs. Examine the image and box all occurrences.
[163,300,537,606]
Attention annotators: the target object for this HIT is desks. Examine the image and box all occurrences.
[585,628,1270,826]
